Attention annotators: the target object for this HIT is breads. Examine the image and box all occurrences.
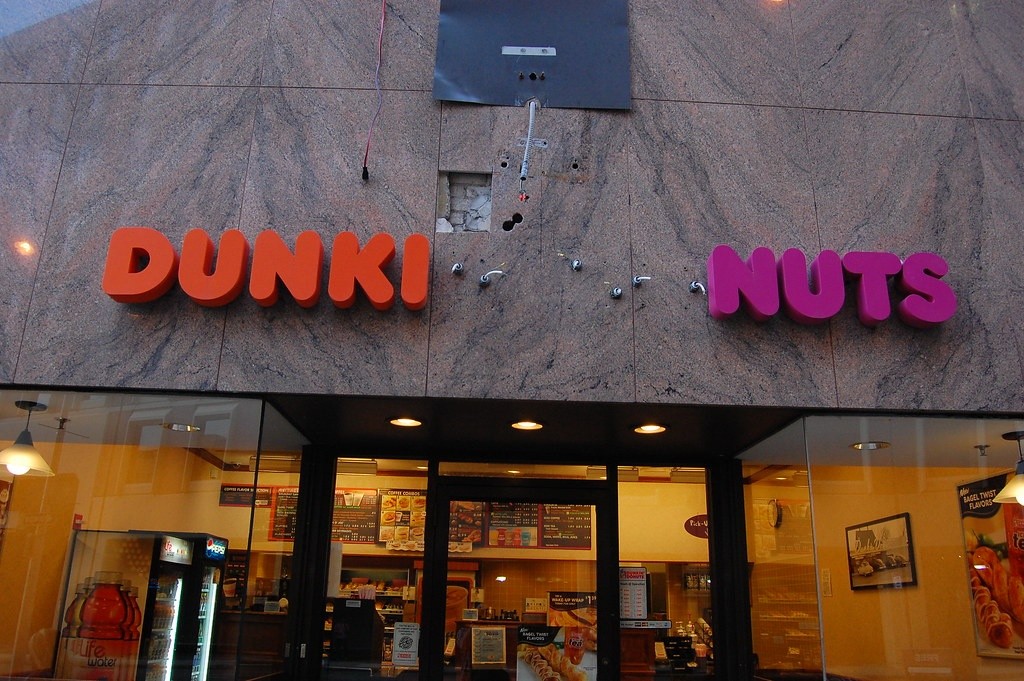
[968,547,1024,648]
[538,644,587,681]
[526,651,560,681]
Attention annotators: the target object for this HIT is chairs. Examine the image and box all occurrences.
[324,599,385,681]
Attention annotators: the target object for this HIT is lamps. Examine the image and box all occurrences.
[0,401,55,476]
[992,431,1024,506]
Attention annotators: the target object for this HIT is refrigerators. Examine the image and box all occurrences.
[55,530,193,681]
[128,530,228,681]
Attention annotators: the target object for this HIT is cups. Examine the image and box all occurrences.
[507,532,512,546]
[396,512,402,522]
[223,578,236,598]
[489,530,498,544]
[565,628,589,665]
[344,492,352,507]
[496,528,507,545]
[354,492,364,507]
[521,531,530,546]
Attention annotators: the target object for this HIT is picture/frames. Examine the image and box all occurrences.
[845,512,917,590]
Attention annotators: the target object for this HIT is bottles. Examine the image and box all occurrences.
[140,576,177,681]
[56,572,140,681]
[191,572,210,681]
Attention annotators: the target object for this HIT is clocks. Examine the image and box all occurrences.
[767,499,782,529]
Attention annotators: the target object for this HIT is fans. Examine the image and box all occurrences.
[38,417,90,440]
